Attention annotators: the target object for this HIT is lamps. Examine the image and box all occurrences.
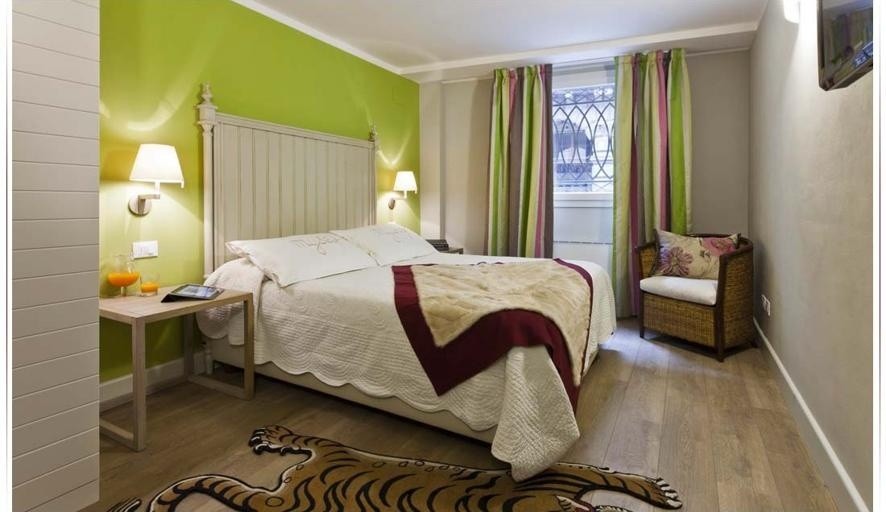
[127,142,184,218]
[387,169,420,209]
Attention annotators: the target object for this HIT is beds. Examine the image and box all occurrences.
[191,79,607,462]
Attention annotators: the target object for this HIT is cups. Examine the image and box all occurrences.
[139,267,161,297]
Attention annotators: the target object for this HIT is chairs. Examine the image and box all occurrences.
[626,231,755,365]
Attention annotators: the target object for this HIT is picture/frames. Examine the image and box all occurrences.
[162,282,224,302]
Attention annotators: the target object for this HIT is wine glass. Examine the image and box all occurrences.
[108,253,139,298]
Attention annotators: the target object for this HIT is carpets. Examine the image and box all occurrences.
[108,421,681,511]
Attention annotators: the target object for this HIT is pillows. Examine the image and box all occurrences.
[648,227,741,278]
[226,220,440,291]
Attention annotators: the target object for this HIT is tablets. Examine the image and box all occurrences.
[168,284,224,300]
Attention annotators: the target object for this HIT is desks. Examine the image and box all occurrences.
[83,282,257,452]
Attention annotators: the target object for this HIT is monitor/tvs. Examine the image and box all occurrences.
[816,0,873,91]
